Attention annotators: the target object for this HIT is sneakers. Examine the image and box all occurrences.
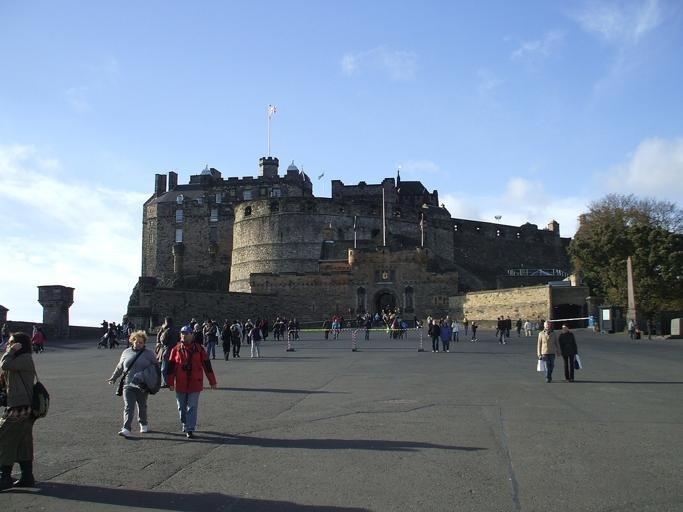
[442,351,445,352]
[432,350,435,352]
[160,385,169,389]
[187,431,193,438]
[546,379,550,382]
[471,338,480,341]
[498,341,508,344]
[140,423,152,432]
[570,379,574,382]
[0,476,13,492]
[118,428,130,436]
[566,380,569,382]
[13,473,35,488]
[436,351,438,352]
[182,424,186,432]
[447,350,449,352]
[208,355,260,361]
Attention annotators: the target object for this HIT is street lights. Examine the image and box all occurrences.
[495,216,502,224]
[420,204,429,245]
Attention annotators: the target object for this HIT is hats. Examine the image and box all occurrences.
[180,326,192,332]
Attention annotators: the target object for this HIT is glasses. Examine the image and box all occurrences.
[6,340,18,346]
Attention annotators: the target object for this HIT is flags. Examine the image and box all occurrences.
[269,105,276,118]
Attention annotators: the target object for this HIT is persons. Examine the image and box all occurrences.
[628,319,635,339]
[0,324,46,354]
[559,324,577,382]
[108,331,161,437]
[537,320,561,383]
[155,316,300,388]
[0,332,35,491]
[323,305,545,352]
[97,320,135,349]
[167,326,217,438]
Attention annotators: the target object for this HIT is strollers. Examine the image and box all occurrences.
[97,332,108,349]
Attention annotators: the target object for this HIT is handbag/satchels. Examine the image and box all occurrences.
[537,360,545,371]
[31,382,49,418]
[574,354,582,369]
[116,381,125,396]
